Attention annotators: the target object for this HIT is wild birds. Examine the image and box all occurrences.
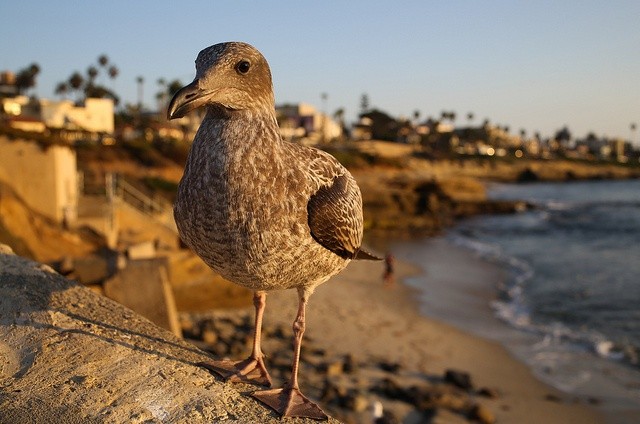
[166,40,384,423]
